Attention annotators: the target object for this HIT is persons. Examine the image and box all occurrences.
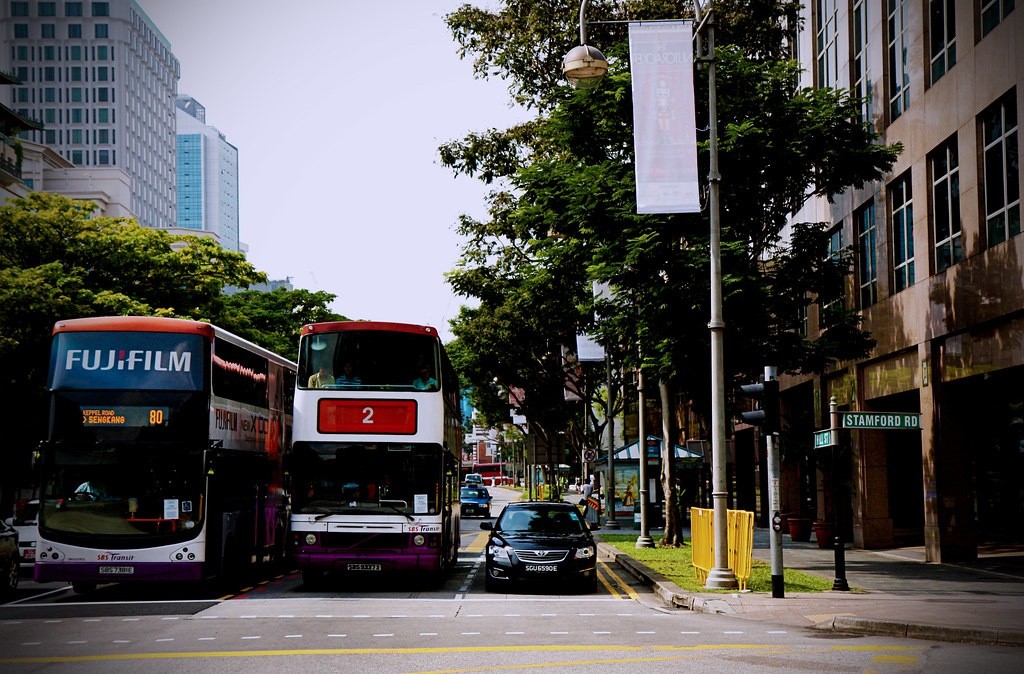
[308,361,335,388]
[413,367,436,389]
[335,363,362,387]
[74,480,106,501]
[578,479,593,517]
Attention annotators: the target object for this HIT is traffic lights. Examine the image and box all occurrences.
[740,381,781,435]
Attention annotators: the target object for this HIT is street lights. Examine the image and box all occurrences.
[562,0,740,589]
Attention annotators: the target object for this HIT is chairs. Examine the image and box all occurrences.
[510,513,532,530]
[552,514,574,526]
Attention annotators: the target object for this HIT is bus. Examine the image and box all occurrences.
[32,316,463,595]
[472,462,513,485]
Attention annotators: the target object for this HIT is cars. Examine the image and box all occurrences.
[480,500,597,592]
[0,499,40,597]
[459,473,492,517]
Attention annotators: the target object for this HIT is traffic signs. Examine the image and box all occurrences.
[813,410,923,449]
